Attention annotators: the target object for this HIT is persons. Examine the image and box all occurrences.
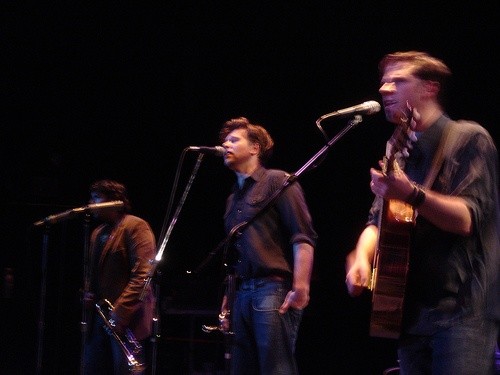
[76,182,156,375]
[213,118,320,375]
[345,51,500,375]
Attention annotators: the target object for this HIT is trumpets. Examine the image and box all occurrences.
[79,288,146,375]
[202,309,234,336]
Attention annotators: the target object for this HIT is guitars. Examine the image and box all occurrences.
[367,101,421,340]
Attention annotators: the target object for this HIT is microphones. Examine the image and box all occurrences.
[323,100,381,120]
[185,145,226,157]
[87,201,124,210]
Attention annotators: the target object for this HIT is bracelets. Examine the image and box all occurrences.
[405,182,426,208]
